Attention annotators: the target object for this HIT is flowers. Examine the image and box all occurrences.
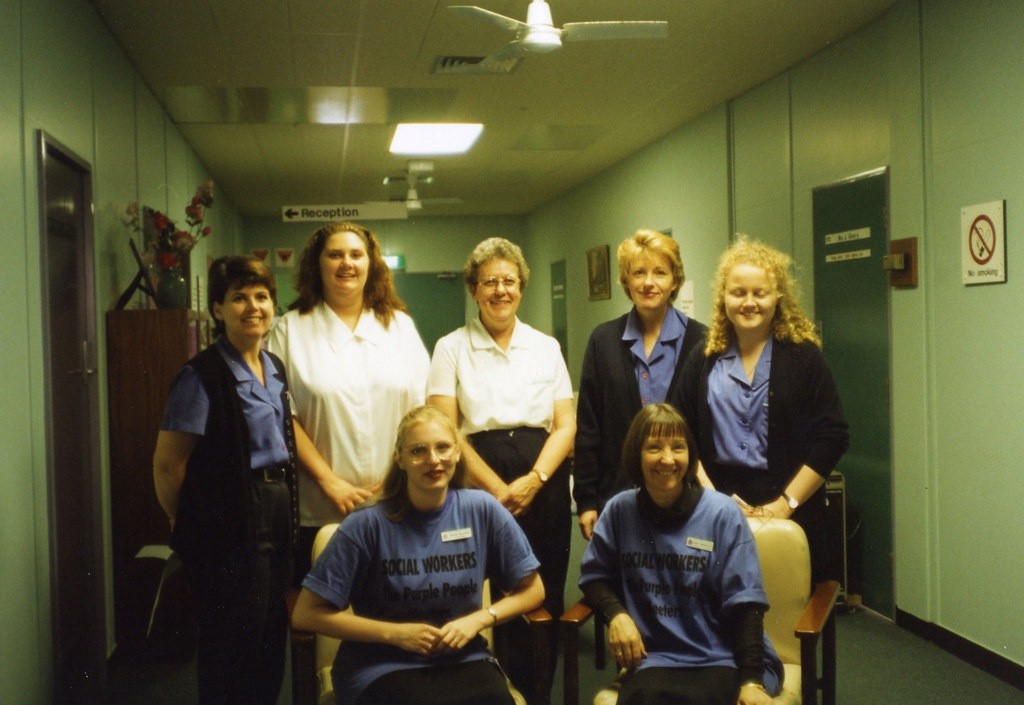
[126,176,216,267]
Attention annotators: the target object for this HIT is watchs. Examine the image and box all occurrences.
[487,607,498,624]
[782,493,800,509]
[532,468,548,483]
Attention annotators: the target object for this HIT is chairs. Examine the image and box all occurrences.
[284,523,554,705]
[558,517,842,705]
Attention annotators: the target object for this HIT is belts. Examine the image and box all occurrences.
[250,466,291,483]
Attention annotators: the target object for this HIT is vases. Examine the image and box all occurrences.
[158,264,187,309]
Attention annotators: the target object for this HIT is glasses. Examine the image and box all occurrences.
[400,441,461,463]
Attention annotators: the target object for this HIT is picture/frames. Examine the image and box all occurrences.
[585,244,612,301]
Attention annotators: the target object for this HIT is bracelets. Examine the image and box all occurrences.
[748,682,765,692]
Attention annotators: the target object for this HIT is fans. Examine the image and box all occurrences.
[445,0,670,77]
[367,172,466,208]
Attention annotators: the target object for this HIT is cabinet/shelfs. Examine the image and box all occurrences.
[106,309,215,673]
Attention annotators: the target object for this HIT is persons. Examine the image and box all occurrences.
[292,406,545,705]
[577,403,784,705]
[427,237,576,705]
[676,233,849,592]
[153,256,301,705]
[274,222,432,705]
[573,229,714,539]
